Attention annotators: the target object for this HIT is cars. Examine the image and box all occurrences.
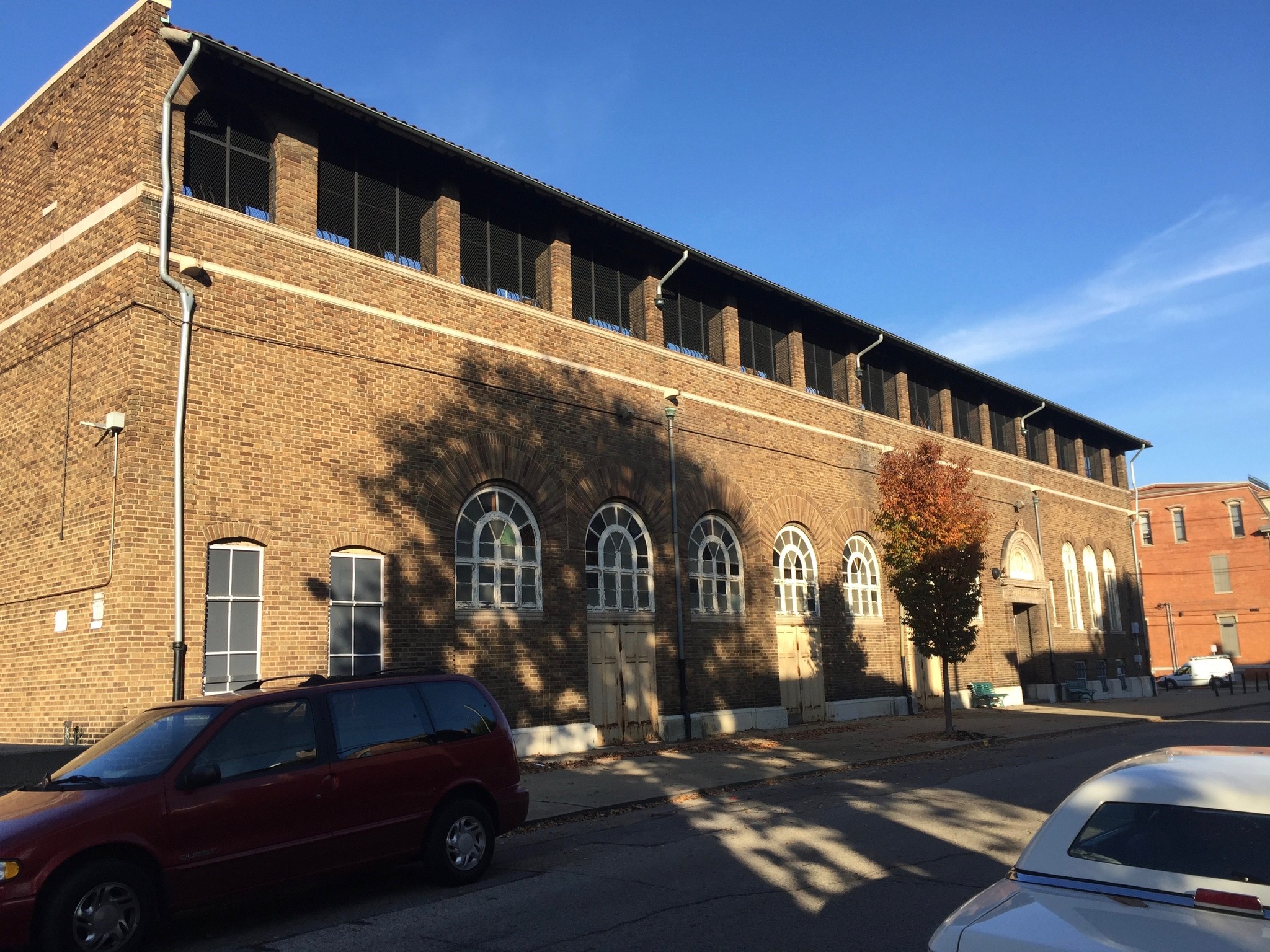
[927,744,1270,952]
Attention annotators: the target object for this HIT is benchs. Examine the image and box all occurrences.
[970,681,1009,708]
[1065,681,1097,703]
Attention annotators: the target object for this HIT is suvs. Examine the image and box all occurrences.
[0,663,531,952]
[1159,654,1235,690]
